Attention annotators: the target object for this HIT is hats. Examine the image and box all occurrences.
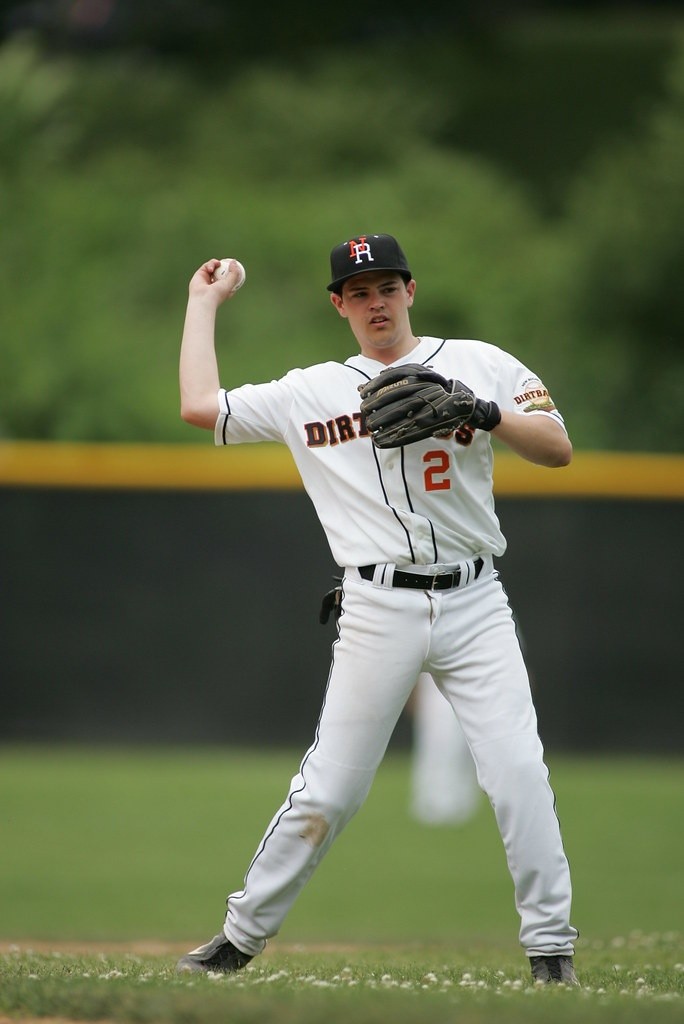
[326,233,412,293]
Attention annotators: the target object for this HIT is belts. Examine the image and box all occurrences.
[358,558,483,592]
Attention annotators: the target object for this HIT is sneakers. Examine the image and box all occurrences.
[529,955,580,987]
[174,932,254,974]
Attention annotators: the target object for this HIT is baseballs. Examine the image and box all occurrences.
[212,255,247,290]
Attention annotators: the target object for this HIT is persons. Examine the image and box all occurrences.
[179,232,584,986]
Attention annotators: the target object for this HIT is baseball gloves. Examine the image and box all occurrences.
[358,360,503,451]
